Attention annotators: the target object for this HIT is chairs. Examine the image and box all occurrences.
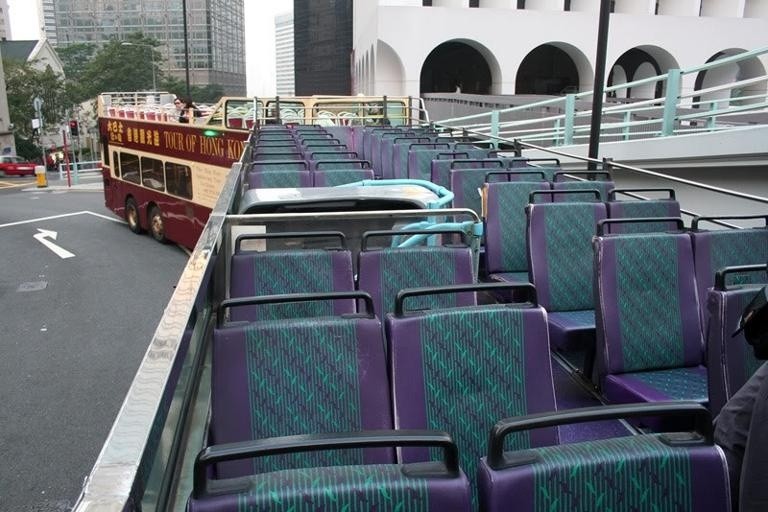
[107,102,768,511]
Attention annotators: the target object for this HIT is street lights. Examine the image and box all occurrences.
[120,41,157,92]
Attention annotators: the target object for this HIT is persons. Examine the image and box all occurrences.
[179,96,203,123]
[711,283,767,512]
[171,98,183,122]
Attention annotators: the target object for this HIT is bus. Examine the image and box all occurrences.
[70,126,768,512]
[98,89,412,251]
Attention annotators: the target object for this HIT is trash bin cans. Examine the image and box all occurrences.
[34,165,48,187]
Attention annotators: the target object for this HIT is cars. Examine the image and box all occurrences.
[0,156,40,179]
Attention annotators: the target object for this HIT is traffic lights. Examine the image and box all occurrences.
[69,120,78,137]
